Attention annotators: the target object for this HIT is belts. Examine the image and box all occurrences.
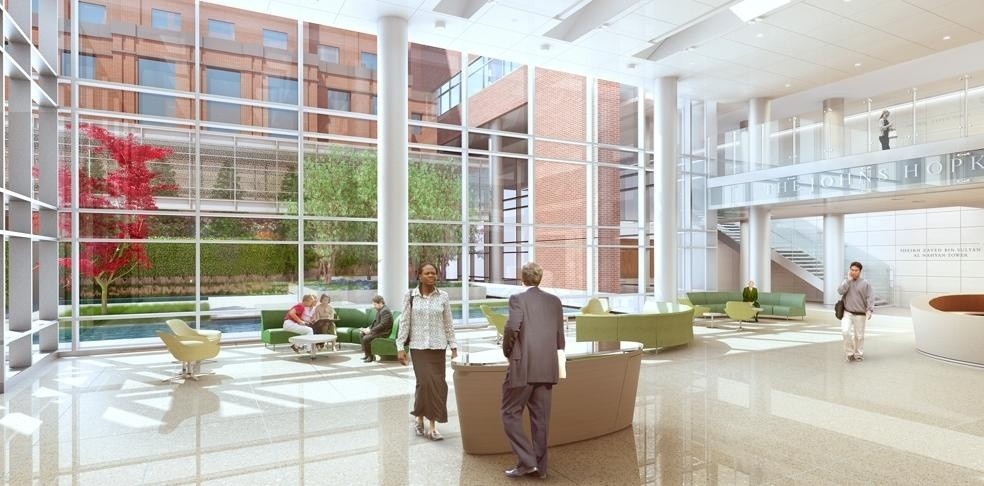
[845,309,866,316]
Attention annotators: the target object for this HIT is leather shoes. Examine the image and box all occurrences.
[505,466,538,476]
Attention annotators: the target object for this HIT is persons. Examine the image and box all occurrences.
[501,262,566,480]
[878,110,897,151]
[282,295,314,353]
[837,261,875,363]
[359,295,394,363]
[741,281,760,309]
[301,294,322,352]
[313,293,339,353]
[395,261,457,442]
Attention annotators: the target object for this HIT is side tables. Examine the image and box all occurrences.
[752,307,764,322]
[703,312,725,329]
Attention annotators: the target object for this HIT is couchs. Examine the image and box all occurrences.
[351,305,409,362]
[259,307,366,350]
[679,290,806,324]
[576,300,694,352]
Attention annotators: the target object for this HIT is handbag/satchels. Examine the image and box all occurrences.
[395,321,411,346]
[835,300,844,319]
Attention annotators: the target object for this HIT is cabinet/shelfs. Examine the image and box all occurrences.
[450,338,643,453]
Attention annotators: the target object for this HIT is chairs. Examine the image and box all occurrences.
[582,297,609,352]
[480,304,508,344]
[165,318,223,376]
[724,301,759,333]
[154,328,219,380]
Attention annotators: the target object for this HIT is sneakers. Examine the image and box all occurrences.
[429,430,443,440]
[414,420,425,435]
